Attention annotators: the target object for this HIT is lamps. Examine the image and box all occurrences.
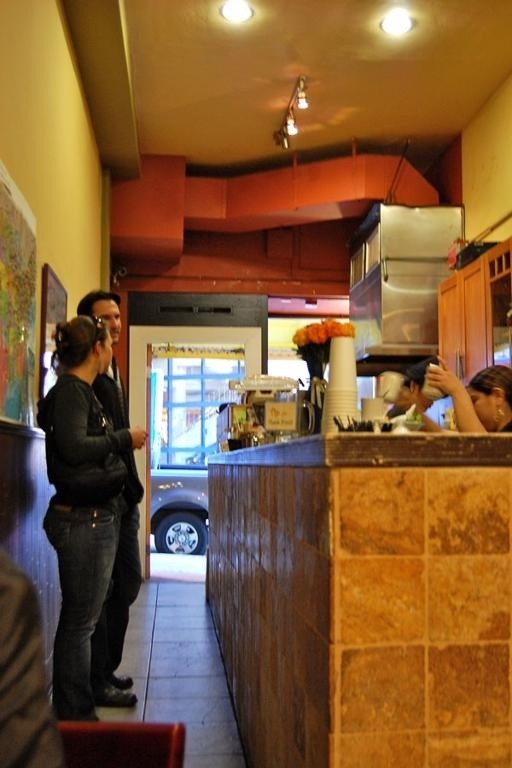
[276,68,310,154]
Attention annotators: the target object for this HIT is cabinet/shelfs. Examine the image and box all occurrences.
[436,255,493,389]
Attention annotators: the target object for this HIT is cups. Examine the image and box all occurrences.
[320,336,359,435]
[379,371,405,403]
[361,398,385,423]
[422,366,443,401]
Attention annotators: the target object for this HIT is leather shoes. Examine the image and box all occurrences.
[66,675,138,722]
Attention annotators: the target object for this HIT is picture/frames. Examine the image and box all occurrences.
[37,263,71,402]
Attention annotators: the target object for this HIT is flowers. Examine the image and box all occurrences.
[291,314,355,401]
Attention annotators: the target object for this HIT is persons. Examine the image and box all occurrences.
[75,288,145,709]
[386,377,434,419]
[38,312,152,722]
[395,354,512,432]
[0,546,69,768]
